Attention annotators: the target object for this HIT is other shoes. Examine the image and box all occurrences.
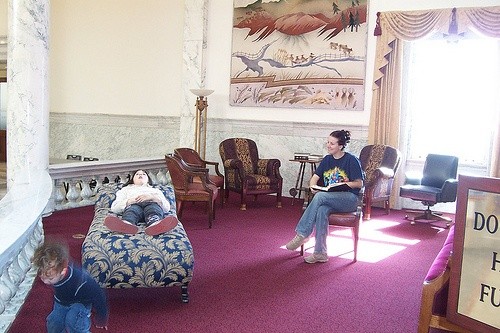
[104,215,138,234]
[145,215,178,236]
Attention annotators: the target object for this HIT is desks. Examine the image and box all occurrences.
[288,160,319,206]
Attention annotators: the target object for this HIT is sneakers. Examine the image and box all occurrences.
[286,233,309,250]
[303,253,327,263]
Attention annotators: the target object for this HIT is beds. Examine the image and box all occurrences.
[80,185,194,301]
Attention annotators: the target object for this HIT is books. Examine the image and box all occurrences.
[311,183,352,192]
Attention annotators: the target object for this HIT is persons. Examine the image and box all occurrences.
[31,244,109,333]
[278,129,365,263]
[105,169,178,235]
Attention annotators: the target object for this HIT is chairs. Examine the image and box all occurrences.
[359,144,401,221]
[300,169,365,263]
[174,148,224,213]
[165,153,221,229]
[399,154,459,229]
[219,138,282,210]
[417,222,477,333]
